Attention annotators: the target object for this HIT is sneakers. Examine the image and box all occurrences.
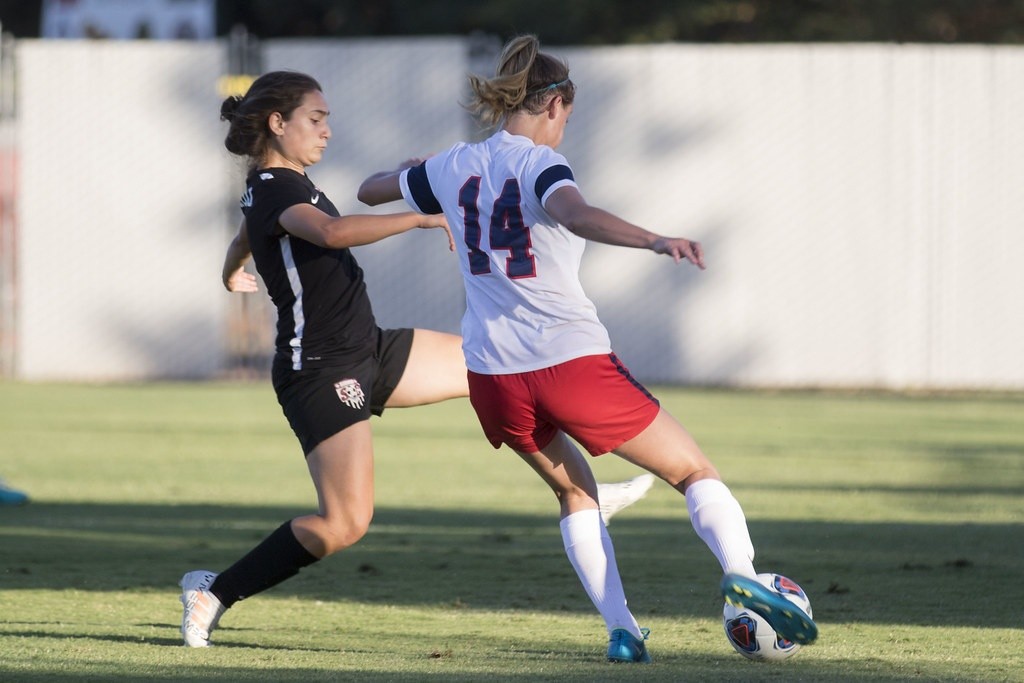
[179,570,226,648]
[606,628,651,663]
[721,573,818,646]
[593,473,656,526]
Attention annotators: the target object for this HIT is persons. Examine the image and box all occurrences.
[175,71,654,649]
[356,38,817,662]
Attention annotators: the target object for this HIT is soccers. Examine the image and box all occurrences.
[723,571,815,663]
